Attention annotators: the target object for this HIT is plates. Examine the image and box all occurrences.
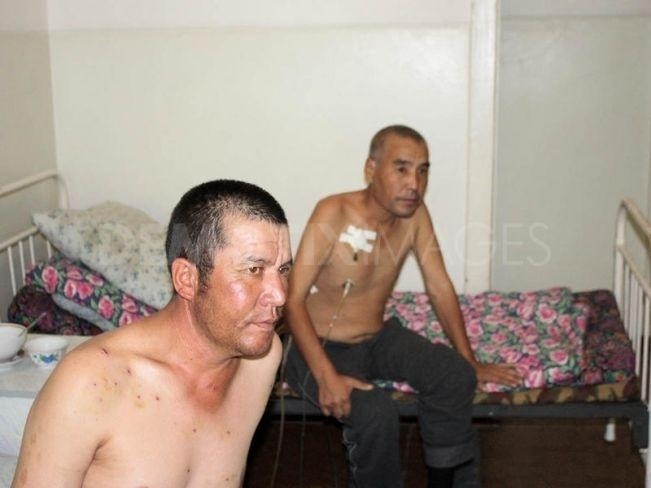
[0,348,26,367]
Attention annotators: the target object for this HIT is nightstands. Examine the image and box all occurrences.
[0,333,94,488]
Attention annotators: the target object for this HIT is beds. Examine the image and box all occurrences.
[0,171,650,465]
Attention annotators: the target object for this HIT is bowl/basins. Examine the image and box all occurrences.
[25,337,69,368]
[0,323,27,361]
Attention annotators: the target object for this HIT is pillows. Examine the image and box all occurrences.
[28,197,175,310]
[30,248,156,333]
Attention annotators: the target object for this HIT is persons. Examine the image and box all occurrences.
[9,178,294,488]
[284,125,525,488]
